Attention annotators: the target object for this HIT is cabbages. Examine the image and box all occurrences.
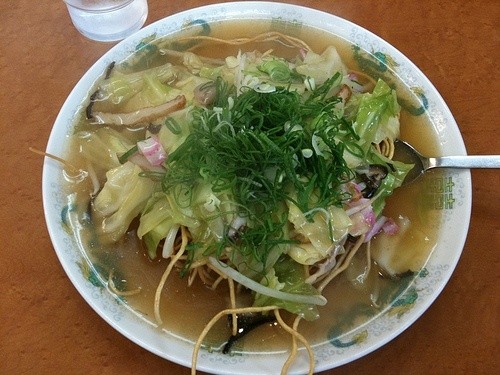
[93,45,415,322]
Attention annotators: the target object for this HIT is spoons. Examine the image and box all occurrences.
[390,135,500,184]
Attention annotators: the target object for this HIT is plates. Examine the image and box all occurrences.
[41,0,472,374]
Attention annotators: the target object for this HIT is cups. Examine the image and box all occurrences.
[63,0,149,42]
